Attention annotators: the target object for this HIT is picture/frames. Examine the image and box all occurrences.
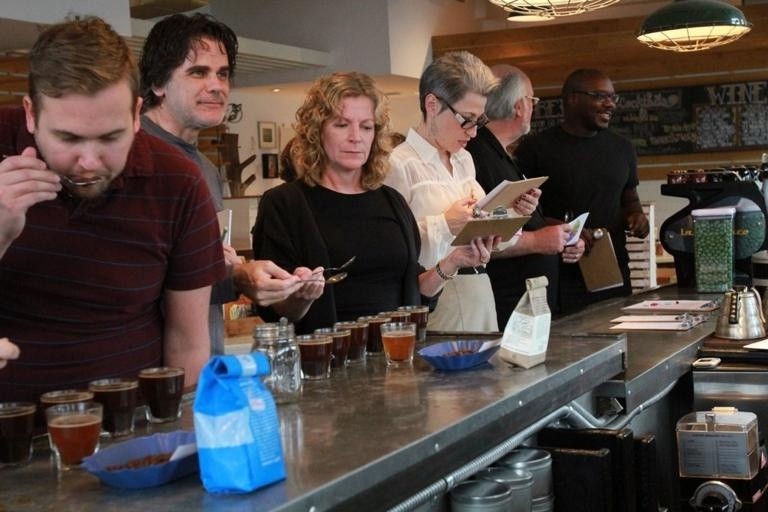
[256,120,278,150]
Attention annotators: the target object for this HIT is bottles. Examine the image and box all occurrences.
[691,207,738,295]
[250,320,304,405]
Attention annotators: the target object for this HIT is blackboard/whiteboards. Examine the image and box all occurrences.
[519,79,768,157]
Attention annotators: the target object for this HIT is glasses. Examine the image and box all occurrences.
[438,96,490,130]
[575,89,619,104]
[526,96,540,105]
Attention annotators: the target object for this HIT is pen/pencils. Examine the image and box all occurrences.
[644,301,679,306]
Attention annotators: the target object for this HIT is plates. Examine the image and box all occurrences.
[81,431,203,491]
[416,339,501,374]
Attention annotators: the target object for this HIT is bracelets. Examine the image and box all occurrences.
[436,263,458,281]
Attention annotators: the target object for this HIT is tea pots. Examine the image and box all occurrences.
[713,285,768,341]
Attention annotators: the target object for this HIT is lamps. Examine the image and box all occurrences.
[634,0,750,54]
[488,1,619,17]
[508,6,556,25]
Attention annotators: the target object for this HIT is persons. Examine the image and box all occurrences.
[278,137,297,182]
[0,337,20,369]
[382,52,543,334]
[251,71,503,334]
[512,68,650,313]
[0,13,228,404]
[136,12,304,356]
[465,65,586,335]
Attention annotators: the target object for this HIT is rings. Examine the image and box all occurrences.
[472,205,482,219]
[576,254,579,259]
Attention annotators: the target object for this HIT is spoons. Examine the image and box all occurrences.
[294,273,348,285]
[311,255,356,275]
[1,153,102,187]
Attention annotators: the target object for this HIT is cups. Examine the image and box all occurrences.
[0,363,185,472]
[297,303,430,383]
